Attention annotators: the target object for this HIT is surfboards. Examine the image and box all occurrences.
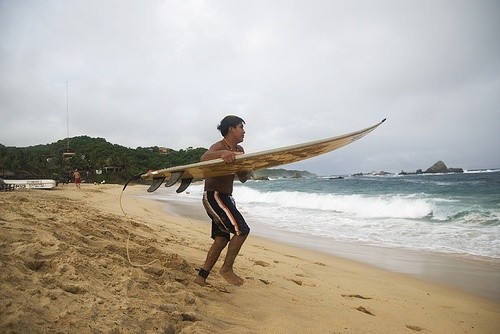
[140,117,387,193]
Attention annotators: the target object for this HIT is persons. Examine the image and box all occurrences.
[193,115,254,287]
[73,168,81,189]
